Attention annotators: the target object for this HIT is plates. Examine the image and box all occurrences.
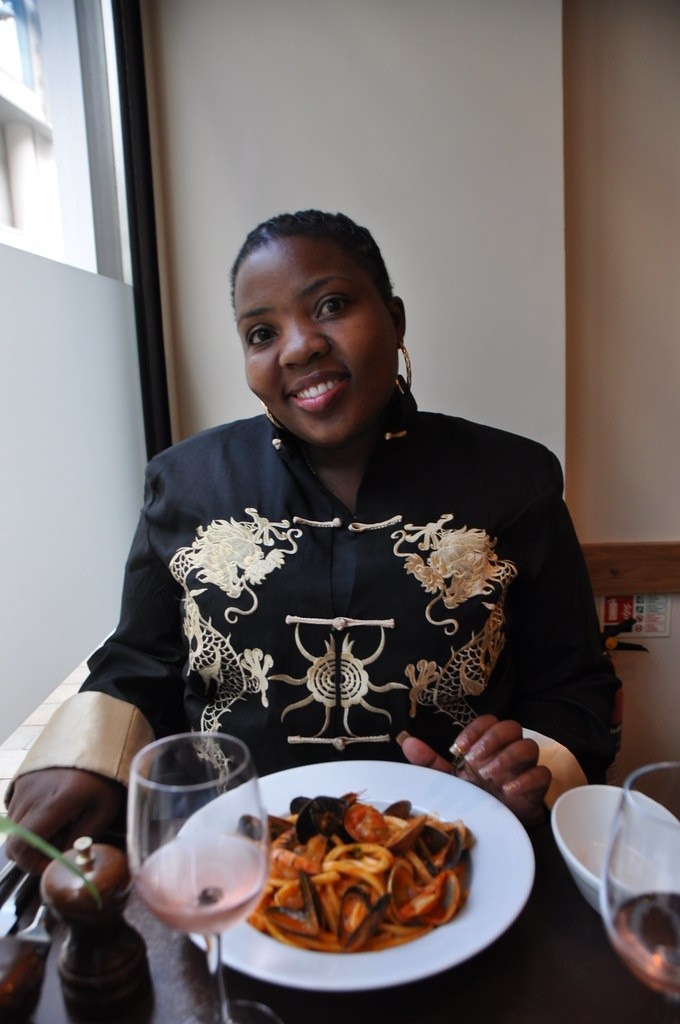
[175,761,536,990]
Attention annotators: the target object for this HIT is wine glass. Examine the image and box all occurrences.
[125,733,280,1024]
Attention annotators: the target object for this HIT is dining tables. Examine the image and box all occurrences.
[0,815,680,1024]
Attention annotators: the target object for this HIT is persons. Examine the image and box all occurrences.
[2,209,619,876]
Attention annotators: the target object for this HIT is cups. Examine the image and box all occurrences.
[602,763,680,1001]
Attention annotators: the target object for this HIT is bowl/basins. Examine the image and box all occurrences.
[552,785,680,916]
